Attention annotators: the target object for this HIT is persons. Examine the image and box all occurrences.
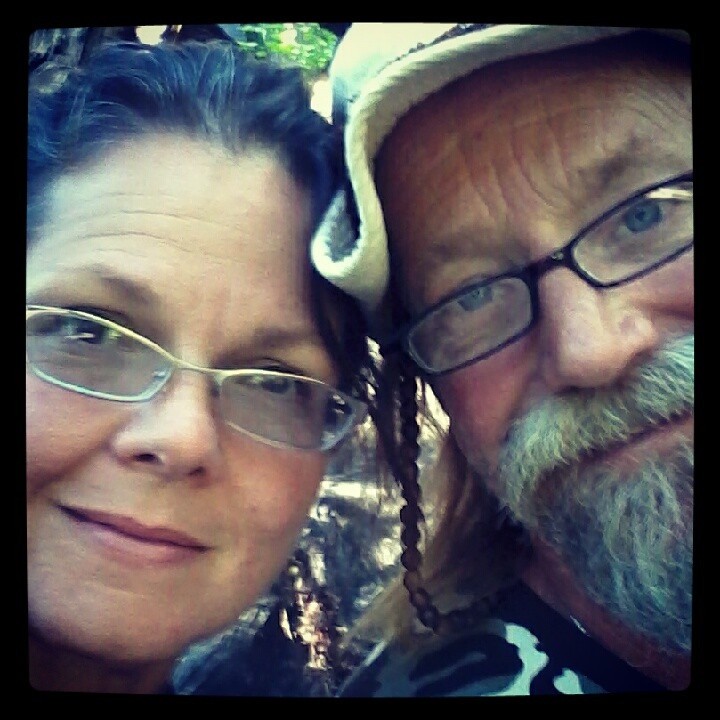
[309,22,694,694]
[27,38,371,694]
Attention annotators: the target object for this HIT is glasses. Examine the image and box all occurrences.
[379,171,694,378]
[23,304,367,455]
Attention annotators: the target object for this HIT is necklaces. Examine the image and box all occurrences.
[568,611,592,637]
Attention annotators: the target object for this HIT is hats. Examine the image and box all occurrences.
[306,24,632,315]
